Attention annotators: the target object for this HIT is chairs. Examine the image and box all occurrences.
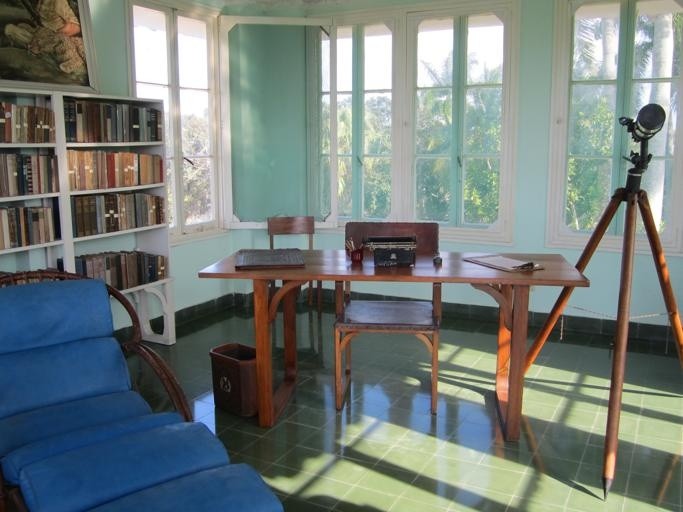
[334,223,439,415]
[268,217,323,352]
[1,273,284,512]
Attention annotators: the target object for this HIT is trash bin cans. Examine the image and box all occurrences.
[206,340,258,418]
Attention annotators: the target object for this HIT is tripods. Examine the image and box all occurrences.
[525,188,683,500]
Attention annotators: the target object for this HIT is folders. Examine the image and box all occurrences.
[462,253,544,273]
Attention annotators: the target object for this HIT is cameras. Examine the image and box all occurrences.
[618,103,666,143]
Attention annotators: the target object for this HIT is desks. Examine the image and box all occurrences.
[199,249,589,442]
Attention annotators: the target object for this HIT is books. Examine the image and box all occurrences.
[0,198,54,249]
[0,94,55,143]
[63,96,163,143]
[56,251,168,291]
[71,193,165,237]
[67,149,163,191]
[0,148,60,196]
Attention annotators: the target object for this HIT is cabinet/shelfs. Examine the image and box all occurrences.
[1,89,176,346]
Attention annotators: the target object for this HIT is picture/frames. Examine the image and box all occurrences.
[0,0,99,94]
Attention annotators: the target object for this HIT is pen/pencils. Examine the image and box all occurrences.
[342,238,365,255]
